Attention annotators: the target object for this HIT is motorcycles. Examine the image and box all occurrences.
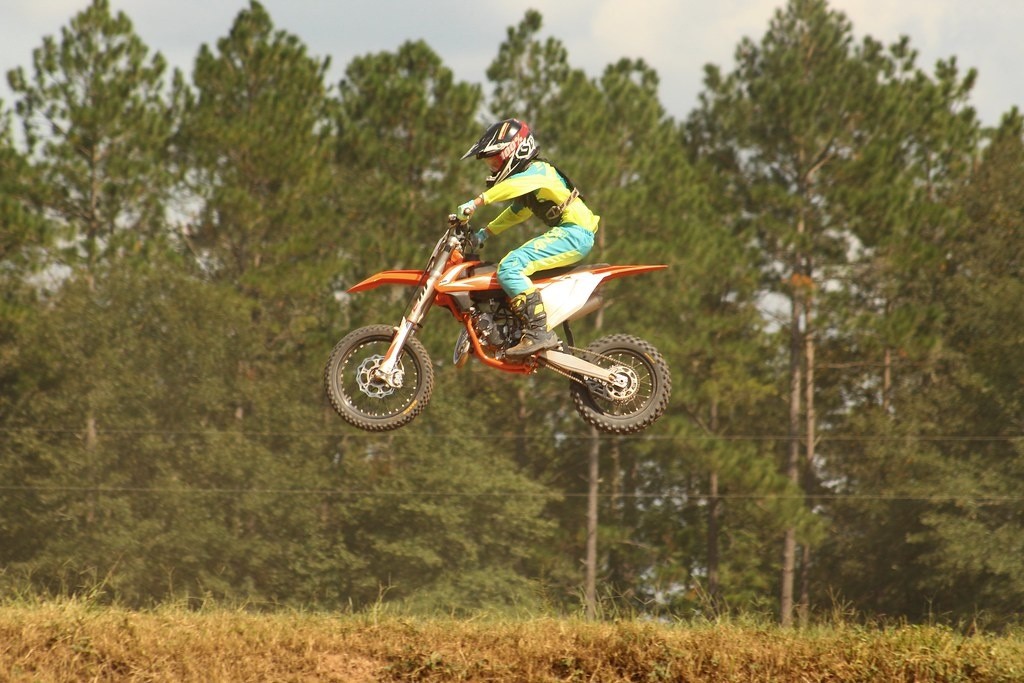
[324,206,673,435]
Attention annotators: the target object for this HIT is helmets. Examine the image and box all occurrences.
[460,118,540,189]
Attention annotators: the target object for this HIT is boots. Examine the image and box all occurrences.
[505,287,558,358]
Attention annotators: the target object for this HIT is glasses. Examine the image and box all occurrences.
[486,154,504,173]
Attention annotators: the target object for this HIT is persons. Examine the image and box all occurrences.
[457,117,602,355]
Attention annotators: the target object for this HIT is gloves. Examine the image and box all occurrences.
[468,228,489,252]
[457,200,476,225]
